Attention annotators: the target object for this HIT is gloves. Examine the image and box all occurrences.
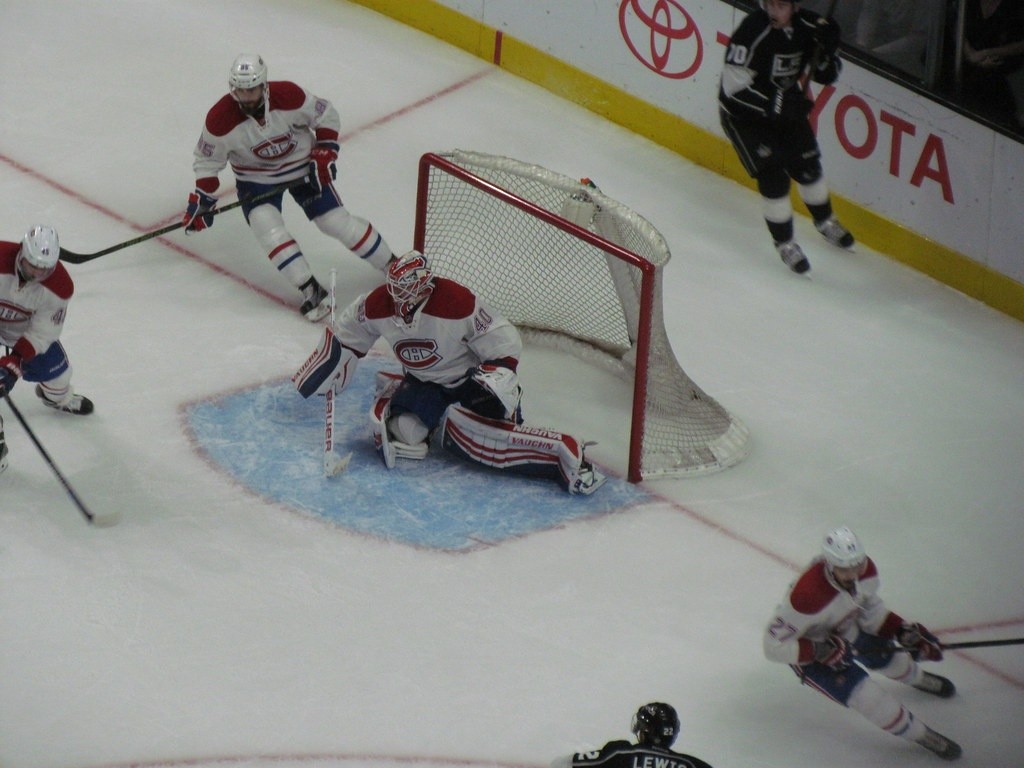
[310,128,339,187]
[896,622,943,663]
[768,89,815,124]
[815,634,854,672]
[0,333,36,399]
[184,177,220,230]
[804,32,832,70]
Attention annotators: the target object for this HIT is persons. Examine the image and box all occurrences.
[801,0,1024,131]
[763,527,961,761]
[0,224,94,473]
[551,702,710,768]
[292,248,607,496]
[182,53,400,320]
[717,0,858,279]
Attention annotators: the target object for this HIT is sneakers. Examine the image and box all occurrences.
[44,394,94,415]
[775,237,810,278]
[815,219,854,252]
[913,726,962,762]
[574,461,608,495]
[299,276,336,322]
[914,670,958,698]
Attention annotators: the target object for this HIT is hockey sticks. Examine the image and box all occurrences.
[322,266,353,479]
[60,174,311,265]
[846,637,1024,660]
[4,394,123,530]
[795,0,838,95]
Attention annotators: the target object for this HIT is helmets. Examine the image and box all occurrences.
[390,250,430,301]
[822,527,868,578]
[632,703,679,748]
[228,53,268,95]
[21,227,61,269]
